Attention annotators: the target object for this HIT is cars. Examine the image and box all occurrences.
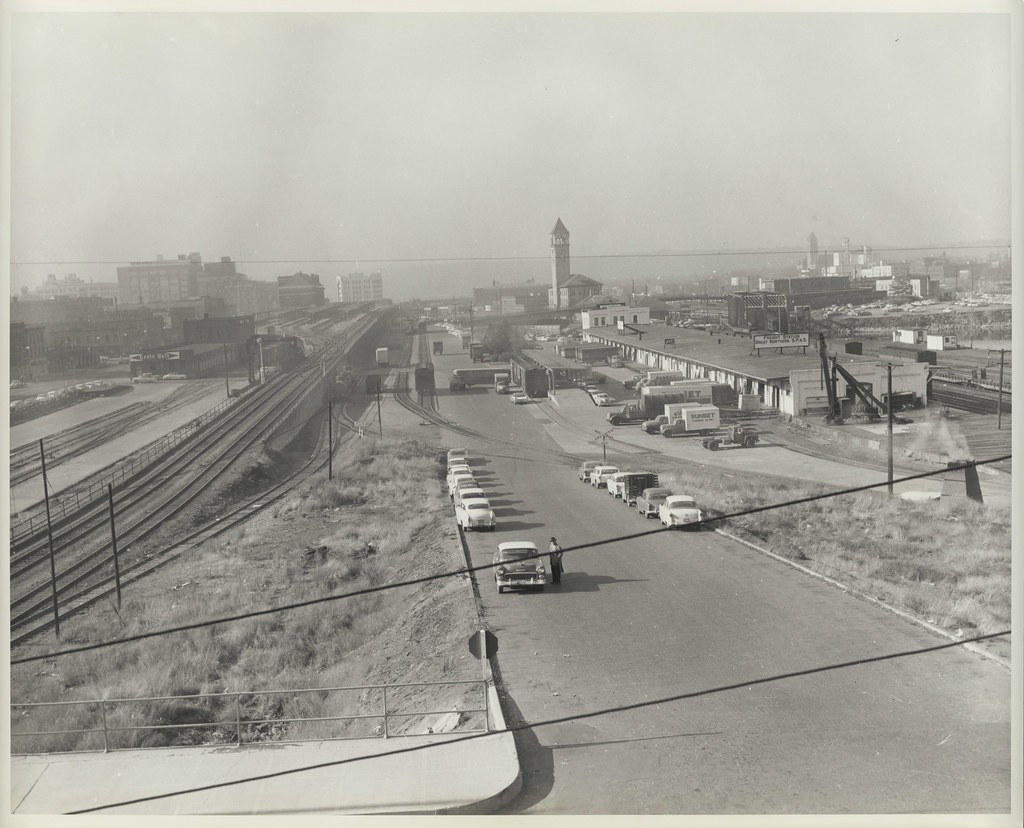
[522,333,574,344]
[579,460,703,530]
[822,299,938,316]
[10,379,111,411]
[950,299,988,307]
[493,542,546,593]
[10,380,23,389]
[574,378,610,406]
[434,322,466,339]
[943,308,956,313]
[446,448,496,532]
[132,373,156,384]
[163,371,186,379]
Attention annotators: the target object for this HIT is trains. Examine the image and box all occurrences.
[418,319,426,333]
[414,361,435,394]
[333,368,355,399]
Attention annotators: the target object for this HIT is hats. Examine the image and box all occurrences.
[550,537,557,542]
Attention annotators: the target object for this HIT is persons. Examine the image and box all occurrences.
[550,537,564,584]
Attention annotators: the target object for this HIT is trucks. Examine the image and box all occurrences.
[605,370,736,436]
[494,373,509,393]
[365,374,381,392]
[470,343,484,363]
[462,335,471,349]
[432,341,443,355]
[376,347,389,366]
[702,426,758,451]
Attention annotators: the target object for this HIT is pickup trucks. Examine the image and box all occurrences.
[511,393,528,405]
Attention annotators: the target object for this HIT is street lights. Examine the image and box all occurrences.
[25,345,30,379]
[96,336,101,364]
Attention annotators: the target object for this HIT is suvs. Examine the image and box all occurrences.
[448,377,465,390]
